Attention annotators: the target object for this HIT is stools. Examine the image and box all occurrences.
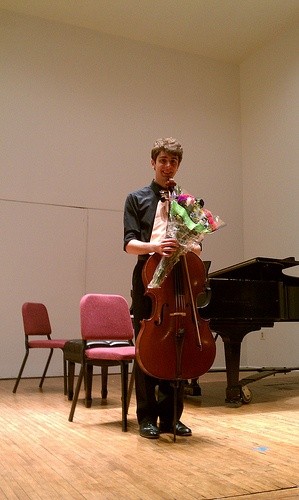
[63,338,133,408]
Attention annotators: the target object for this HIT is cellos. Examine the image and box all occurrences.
[135,190,217,444]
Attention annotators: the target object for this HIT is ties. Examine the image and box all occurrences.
[147,190,169,255]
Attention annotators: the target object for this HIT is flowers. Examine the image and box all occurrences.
[147,176,229,289]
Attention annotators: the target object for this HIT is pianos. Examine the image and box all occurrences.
[197,255,299,407]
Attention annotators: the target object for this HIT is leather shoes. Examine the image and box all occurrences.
[159,419,191,435]
[139,422,160,438]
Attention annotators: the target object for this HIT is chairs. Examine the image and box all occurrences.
[12,302,70,395]
[68,294,136,434]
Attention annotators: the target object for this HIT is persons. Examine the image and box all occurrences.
[123,137,202,439]
